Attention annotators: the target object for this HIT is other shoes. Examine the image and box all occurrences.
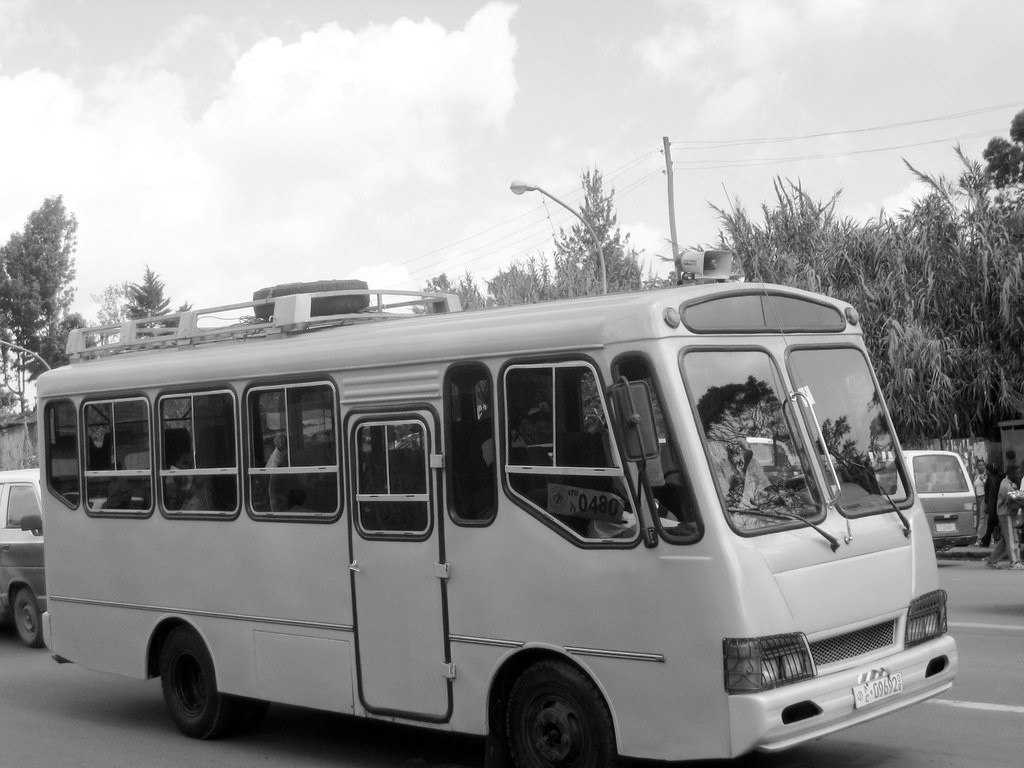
[990,538,995,544]
[974,539,981,546]
[979,543,989,547]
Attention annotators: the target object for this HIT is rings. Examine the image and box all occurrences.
[280,444,281,446]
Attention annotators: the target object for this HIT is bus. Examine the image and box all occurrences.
[37,281,958,768]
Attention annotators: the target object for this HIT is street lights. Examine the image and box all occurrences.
[512,179,609,296]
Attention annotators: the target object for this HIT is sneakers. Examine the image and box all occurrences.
[983,556,999,568]
[1008,562,1024,570]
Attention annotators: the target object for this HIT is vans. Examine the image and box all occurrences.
[744,435,980,554]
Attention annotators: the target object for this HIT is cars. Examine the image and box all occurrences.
[0,466,144,649]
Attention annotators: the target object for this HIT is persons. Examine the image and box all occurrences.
[707,395,775,526]
[973,450,1024,569]
[467,412,548,519]
[588,478,680,538]
[103,431,286,511]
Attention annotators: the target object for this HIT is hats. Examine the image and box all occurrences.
[985,463,998,475]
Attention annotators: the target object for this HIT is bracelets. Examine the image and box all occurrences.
[657,504,668,517]
